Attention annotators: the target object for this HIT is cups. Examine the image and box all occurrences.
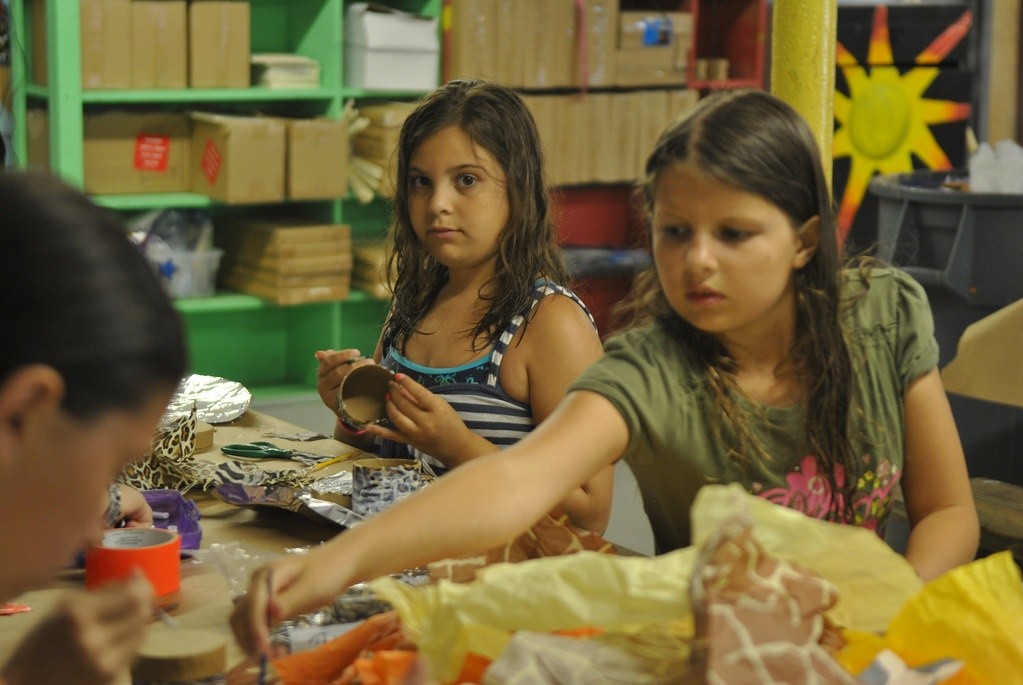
[337,365,402,431]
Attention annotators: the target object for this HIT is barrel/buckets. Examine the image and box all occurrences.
[870,168,1022,488]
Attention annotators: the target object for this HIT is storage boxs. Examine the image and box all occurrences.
[218,218,353,307]
[343,0,439,90]
[143,248,225,298]
[444,0,693,86]
[357,90,699,188]
[26,108,350,203]
[26,0,252,89]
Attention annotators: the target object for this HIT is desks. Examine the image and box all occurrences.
[1,406,651,685]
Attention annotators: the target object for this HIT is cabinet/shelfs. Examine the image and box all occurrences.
[7,0,838,400]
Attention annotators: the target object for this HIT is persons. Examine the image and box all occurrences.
[226,88,980,656]
[0,163,189,684]
[314,78,613,534]
